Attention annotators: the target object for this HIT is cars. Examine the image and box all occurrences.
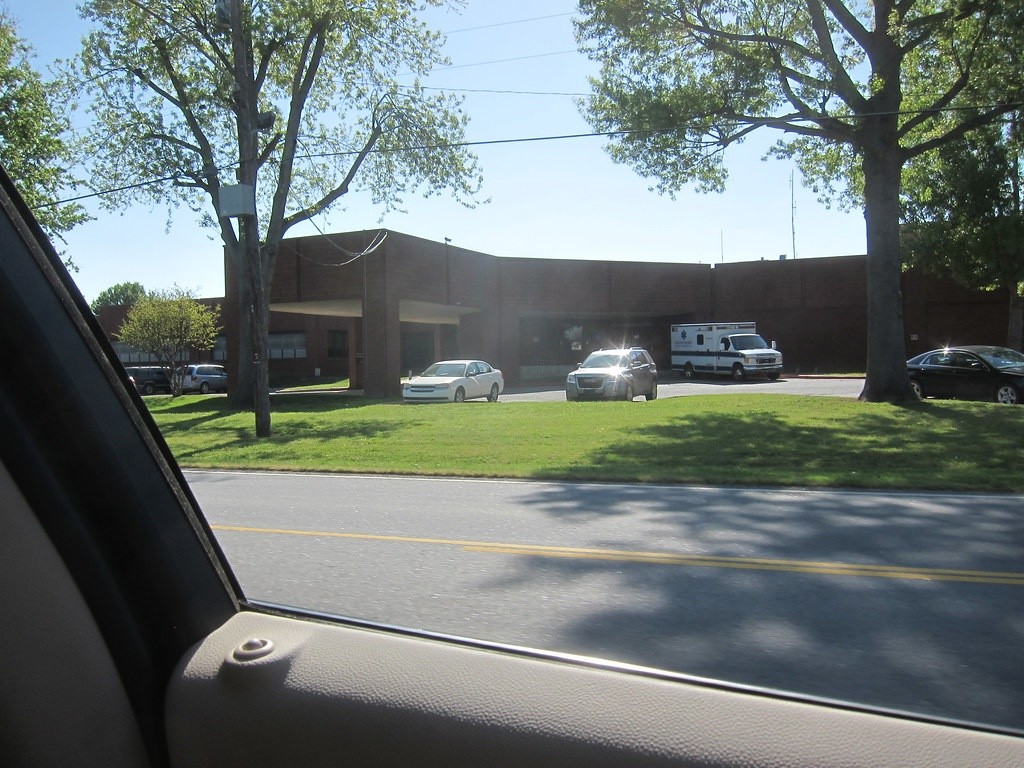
[906,344,1024,406]
[402,359,504,405]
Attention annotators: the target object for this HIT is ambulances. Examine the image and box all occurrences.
[669,322,783,383]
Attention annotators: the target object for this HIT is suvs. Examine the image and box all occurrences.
[125,365,174,395]
[170,364,227,394]
[565,344,657,402]
